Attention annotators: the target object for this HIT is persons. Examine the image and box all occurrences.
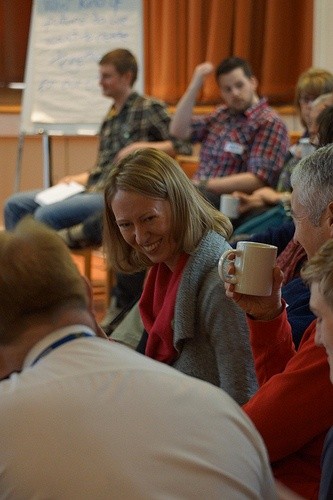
[97,54,333,500]
[3,50,194,232]
[0,229,276,500]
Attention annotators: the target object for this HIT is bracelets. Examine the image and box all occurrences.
[198,175,209,194]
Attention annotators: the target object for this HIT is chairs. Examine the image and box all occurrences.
[70,156,199,310]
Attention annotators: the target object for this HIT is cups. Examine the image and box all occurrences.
[218,242,278,296]
[219,194,241,220]
